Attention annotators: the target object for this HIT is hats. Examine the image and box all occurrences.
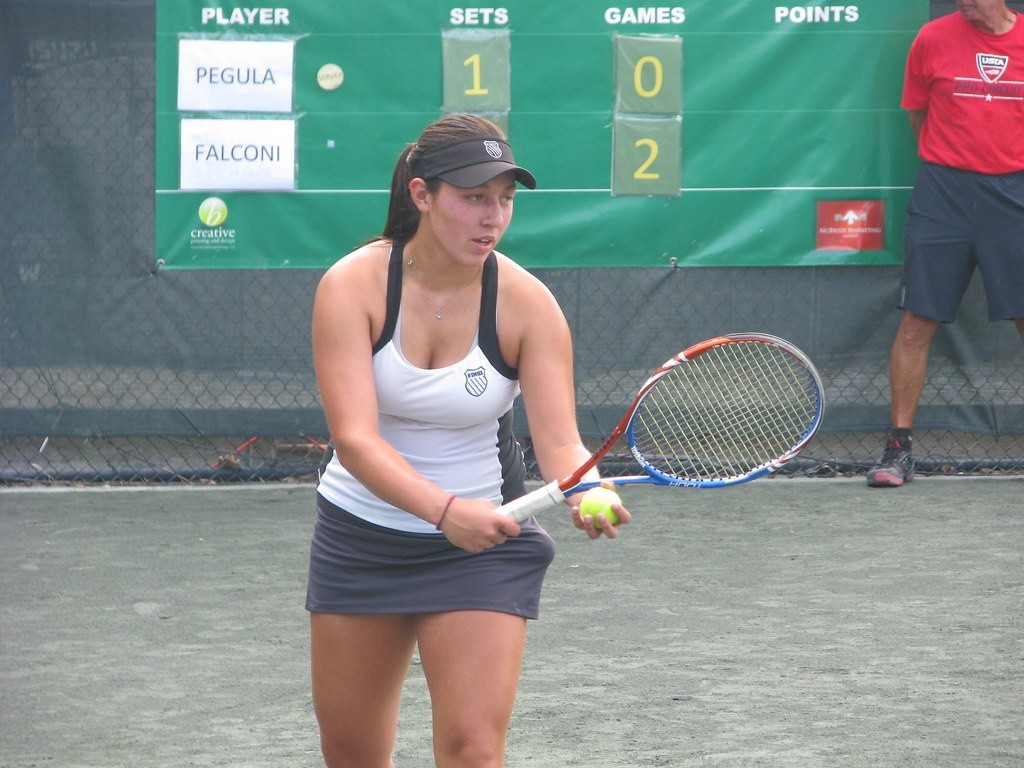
[412,136,535,188]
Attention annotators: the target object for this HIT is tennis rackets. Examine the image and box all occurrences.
[448,331,825,553]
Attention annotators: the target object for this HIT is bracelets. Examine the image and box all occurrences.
[434,494,456,532]
[576,497,583,506]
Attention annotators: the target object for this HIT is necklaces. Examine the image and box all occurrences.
[405,242,465,320]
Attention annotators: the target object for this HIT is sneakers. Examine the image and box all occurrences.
[867,437,914,486]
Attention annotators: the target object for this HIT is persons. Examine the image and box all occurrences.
[866,0,1024,485]
[304,112,633,768]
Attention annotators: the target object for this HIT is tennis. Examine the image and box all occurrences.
[580,486,622,529]
